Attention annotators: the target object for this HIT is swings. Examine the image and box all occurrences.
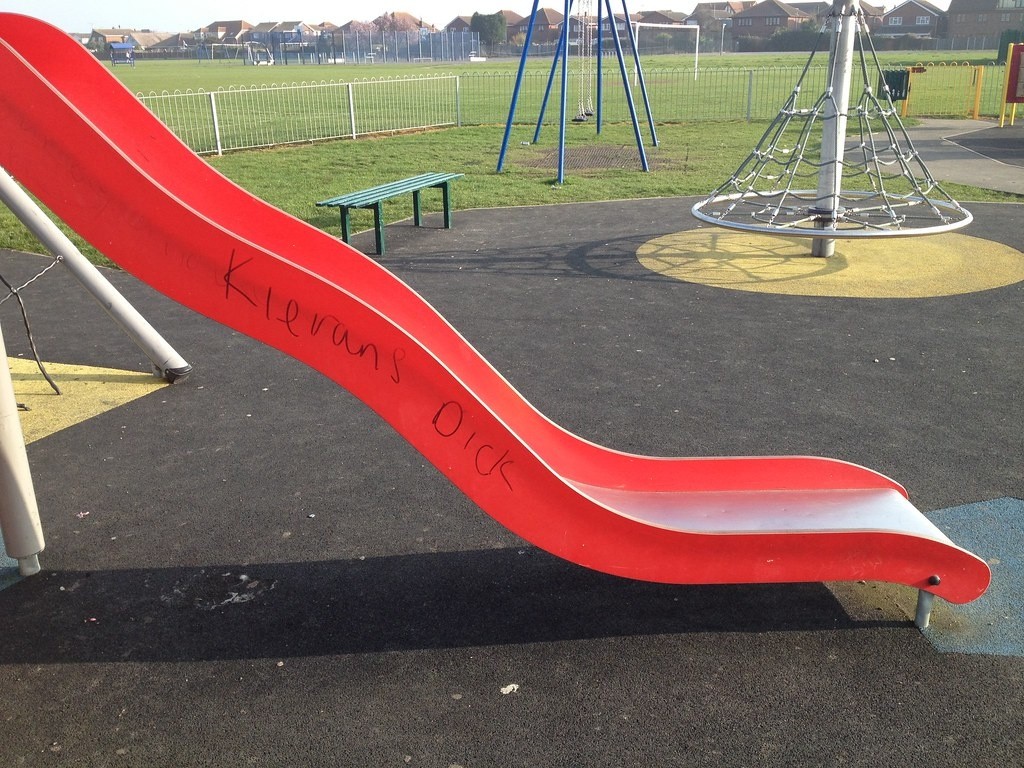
[570,0,595,122]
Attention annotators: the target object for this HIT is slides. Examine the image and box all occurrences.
[1,12,992,605]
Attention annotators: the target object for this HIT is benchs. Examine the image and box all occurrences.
[313,172,466,254]
